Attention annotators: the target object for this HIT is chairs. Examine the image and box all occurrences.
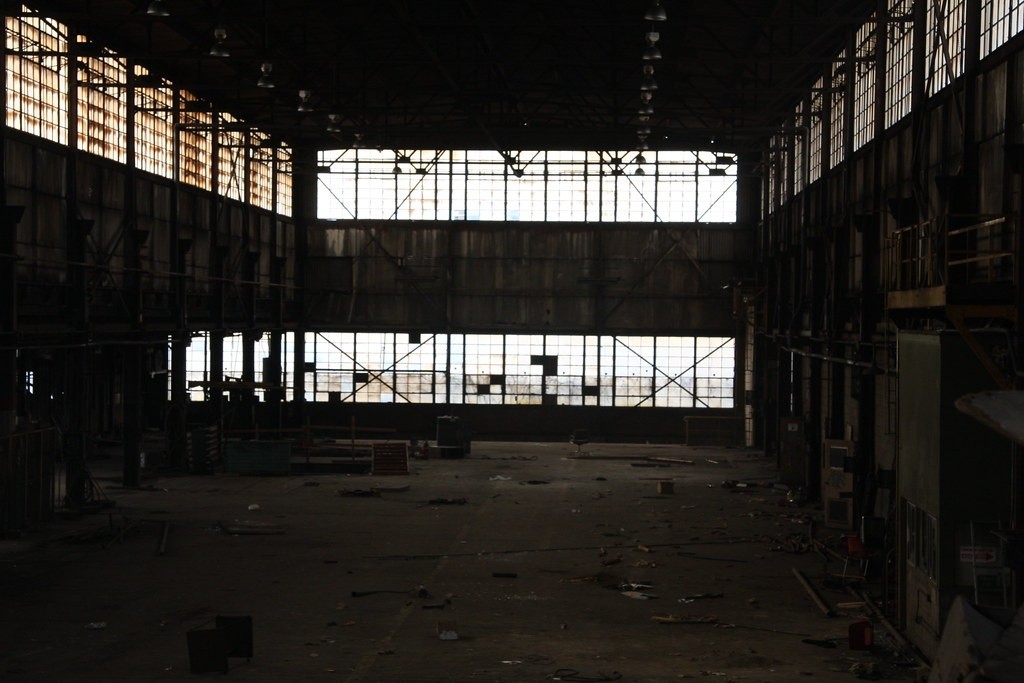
[571,428,595,454]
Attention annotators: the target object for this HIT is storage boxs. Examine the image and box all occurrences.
[657,482,675,494]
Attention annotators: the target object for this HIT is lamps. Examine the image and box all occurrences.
[352,133,365,148]
[636,135,649,150]
[636,115,652,134]
[642,25,662,61]
[146,0,171,17]
[298,90,315,113]
[256,41,276,87]
[638,91,654,114]
[640,63,658,91]
[644,0,667,22]
[326,115,341,132]
[209,17,230,57]
